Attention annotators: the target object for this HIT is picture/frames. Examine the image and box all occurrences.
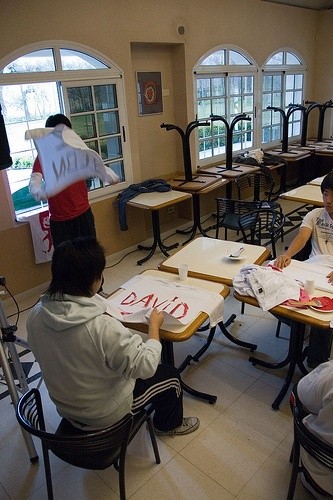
[135,70,165,117]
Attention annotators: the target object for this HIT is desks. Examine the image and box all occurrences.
[233,258,333,410]
[166,178,230,246]
[279,185,324,245]
[104,268,230,418]
[117,190,192,266]
[157,235,271,362]
[160,100,333,192]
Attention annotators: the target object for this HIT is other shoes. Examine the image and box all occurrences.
[303,356,313,372]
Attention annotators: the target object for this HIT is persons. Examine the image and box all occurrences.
[298,360,333,498]
[26,238,199,436]
[274,173,333,285]
[29,115,96,248]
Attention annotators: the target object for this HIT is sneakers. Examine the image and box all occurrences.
[152,417,200,436]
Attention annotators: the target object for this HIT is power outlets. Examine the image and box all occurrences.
[168,206,175,214]
[0,276,7,286]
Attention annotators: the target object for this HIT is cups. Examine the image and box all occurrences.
[178,264,188,281]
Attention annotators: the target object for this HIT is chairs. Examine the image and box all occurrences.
[237,209,286,259]
[286,384,333,500]
[15,388,161,500]
[234,172,282,236]
[214,196,262,245]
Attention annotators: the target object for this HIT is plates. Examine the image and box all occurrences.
[310,294,332,312]
